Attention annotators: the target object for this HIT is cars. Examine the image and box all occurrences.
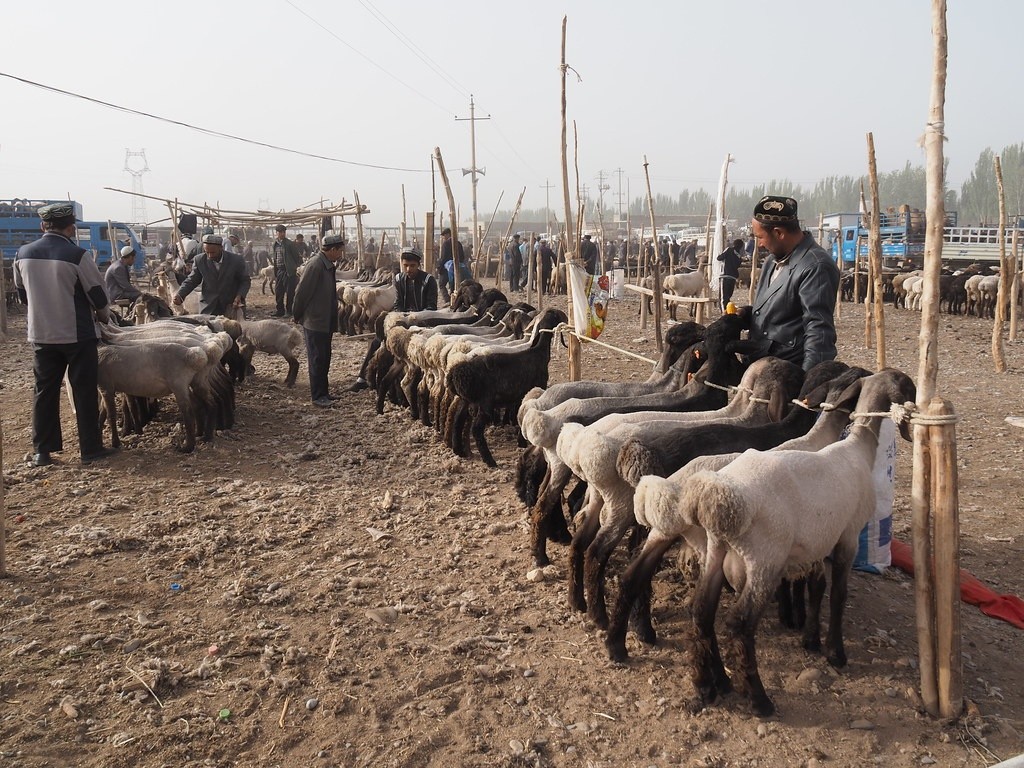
[515,221,714,246]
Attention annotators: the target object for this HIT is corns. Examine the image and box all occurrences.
[727,302,737,314]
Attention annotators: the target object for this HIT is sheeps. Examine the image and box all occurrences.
[471,255,499,277]
[548,263,567,295]
[860,204,926,233]
[750,268,761,284]
[840,261,923,311]
[638,256,675,315]
[88,291,241,453]
[938,263,1024,319]
[941,223,1024,244]
[0,198,48,217]
[682,366,916,717]
[514,305,805,628]
[633,365,874,672]
[334,259,398,342]
[356,279,568,467]
[606,361,852,663]
[860,234,894,244]
[662,256,708,321]
[260,265,276,295]
[226,300,302,388]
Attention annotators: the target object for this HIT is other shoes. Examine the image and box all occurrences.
[313,396,335,408]
[346,377,368,393]
[328,391,342,402]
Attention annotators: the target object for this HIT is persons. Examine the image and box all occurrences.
[347,247,439,393]
[12,203,111,466]
[437,228,464,308]
[508,233,755,299]
[271,225,303,317]
[175,225,375,277]
[291,235,345,407]
[174,234,251,315]
[461,244,476,265]
[104,246,142,325]
[717,239,744,315]
[723,196,842,375]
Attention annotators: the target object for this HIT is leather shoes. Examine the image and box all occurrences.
[35,452,52,466]
[81,446,117,463]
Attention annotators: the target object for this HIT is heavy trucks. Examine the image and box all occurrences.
[832,211,1024,270]
[1,200,147,278]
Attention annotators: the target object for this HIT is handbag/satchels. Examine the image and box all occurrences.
[567,260,609,345]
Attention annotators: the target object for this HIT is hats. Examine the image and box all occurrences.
[180,234,194,239]
[38,202,74,221]
[539,240,545,244]
[320,234,349,246]
[275,224,287,230]
[203,233,222,245]
[440,228,452,234]
[753,194,799,224]
[230,233,239,241]
[120,246,133,258]
[512,234,519,237]
[584,235,591,238]
[401,246,423,258]
